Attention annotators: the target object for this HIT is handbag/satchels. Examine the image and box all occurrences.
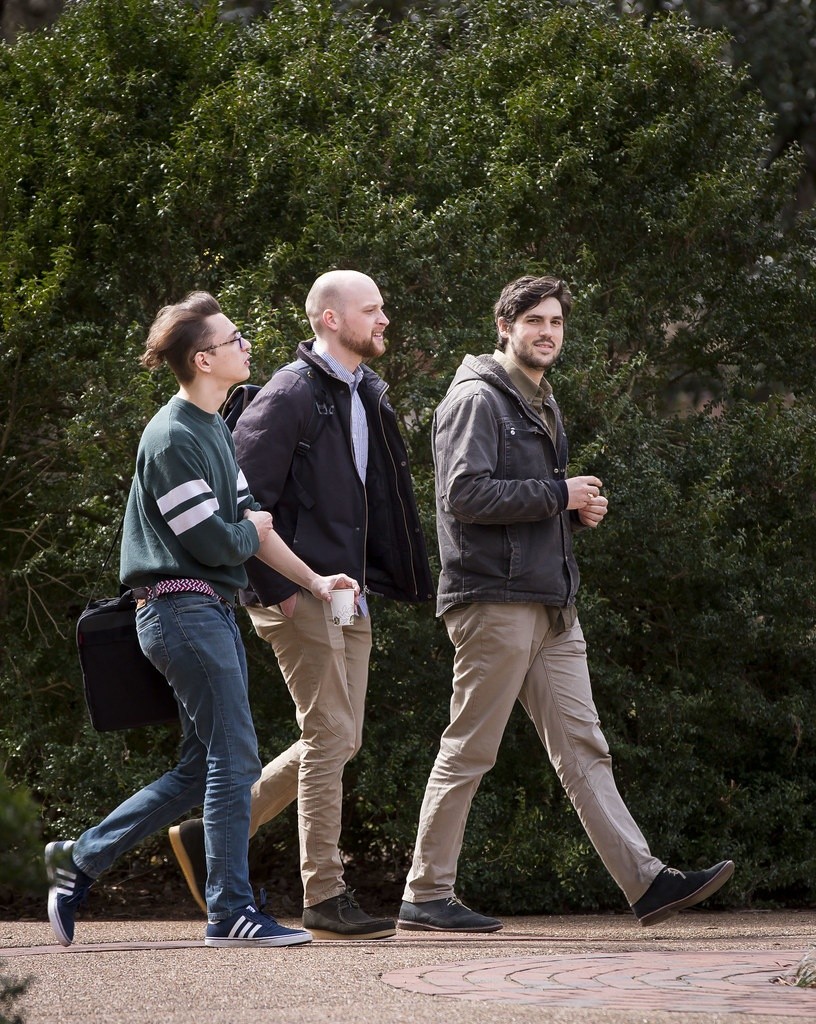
[78,595,180,730]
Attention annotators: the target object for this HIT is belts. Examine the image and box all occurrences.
[146,579,223,600]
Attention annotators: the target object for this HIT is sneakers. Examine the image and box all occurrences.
[631,859,734,925]
[203,907,313,947]
[169,816,211,916]
[45,838,89,947]
[398,899,503,932]
[304,889,396,943]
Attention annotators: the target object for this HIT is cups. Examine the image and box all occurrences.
[327,586,356,627]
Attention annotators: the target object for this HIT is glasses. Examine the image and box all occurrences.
[192,334,244,361]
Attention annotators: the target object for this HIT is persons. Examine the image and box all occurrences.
[43,288,362,950]
[165,270,440,941]
[394,273,736,934]
